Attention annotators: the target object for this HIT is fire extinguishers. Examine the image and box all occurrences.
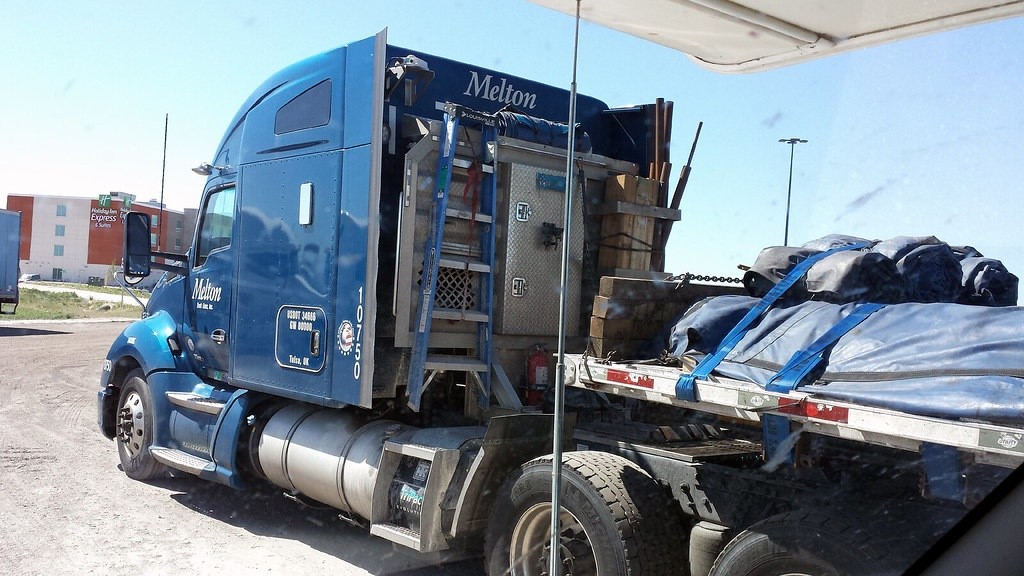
[523,343,549,405]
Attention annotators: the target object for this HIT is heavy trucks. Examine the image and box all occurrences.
[98,26,1023,575]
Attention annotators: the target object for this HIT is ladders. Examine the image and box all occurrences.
[406,100,499,413]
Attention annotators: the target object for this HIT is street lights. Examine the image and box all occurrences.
[779,136,809,249]
